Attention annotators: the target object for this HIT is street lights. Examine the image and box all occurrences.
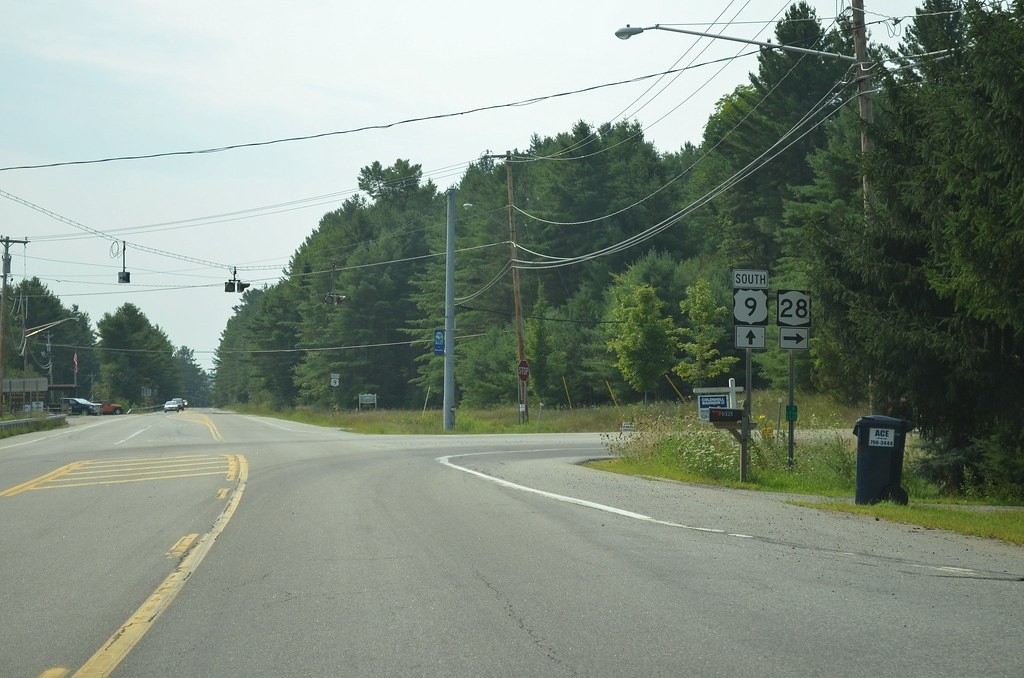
[615,0,883,416]
[22,317,80,377]
[463,203,529,426]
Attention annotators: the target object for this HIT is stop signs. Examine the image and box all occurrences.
[518,360,530,382]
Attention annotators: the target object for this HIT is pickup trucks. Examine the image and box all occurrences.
[92,400,124,415]
[43,398,103,416]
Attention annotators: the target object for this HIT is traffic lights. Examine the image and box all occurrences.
[225,282,234,293]
[324,295,335,305]
[335,294,346,305]
[237,282,250,293]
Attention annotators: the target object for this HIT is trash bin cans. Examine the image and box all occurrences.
[852,412,919,508]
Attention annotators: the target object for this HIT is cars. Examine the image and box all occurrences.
[172,398,185,411]
[162,401,179,413]
[183,400,188,407]
